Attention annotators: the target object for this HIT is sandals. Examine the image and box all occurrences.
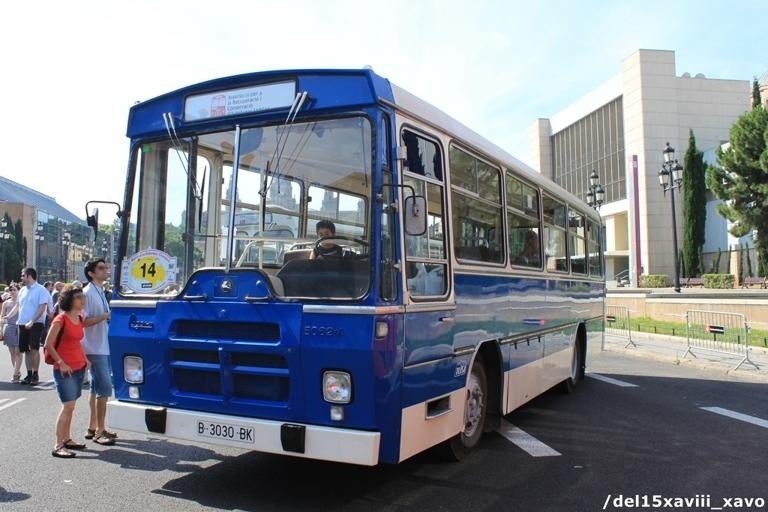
[84,428,118,444]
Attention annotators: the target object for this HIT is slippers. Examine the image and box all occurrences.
[52,445,75,457]
[63,439,85,449]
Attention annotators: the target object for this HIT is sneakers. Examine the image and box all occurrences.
[21,376,39,385]
[13,373,21,384]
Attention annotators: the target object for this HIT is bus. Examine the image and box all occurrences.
[84,65,609,469]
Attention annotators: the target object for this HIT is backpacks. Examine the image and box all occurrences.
[43,314,65,365]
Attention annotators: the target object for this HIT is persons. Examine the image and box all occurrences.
[309,220,344,260]
[43,285,85,457]
[511,229,540,267]
[0,267,83,384]
[83,257,119,445]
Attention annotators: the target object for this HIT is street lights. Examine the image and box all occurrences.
[586,170,605,210]
[61,229,71,283]
[100,238,109,261]
[34,221,45,284]
[658,142,684,292]
[0,216,10,252]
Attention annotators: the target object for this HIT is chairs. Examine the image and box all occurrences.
[283,242,357,264]
